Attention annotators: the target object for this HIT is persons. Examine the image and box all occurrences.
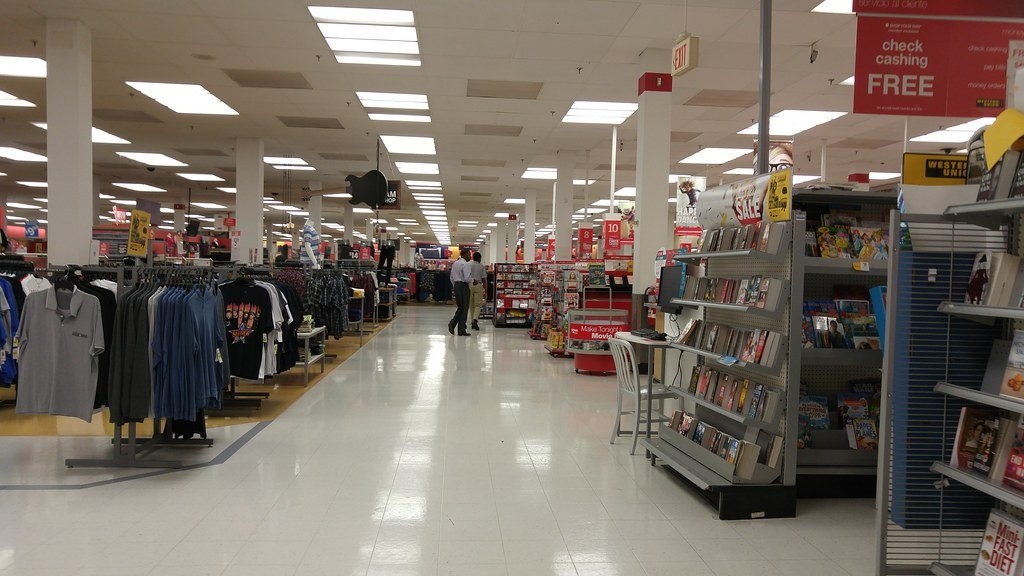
[469,252,487,330]
[448,249,478,336]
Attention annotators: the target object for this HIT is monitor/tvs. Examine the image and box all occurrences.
[657,265,687,315]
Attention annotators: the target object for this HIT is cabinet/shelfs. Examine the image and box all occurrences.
[791,184,898,501]
[927,191,1024,576]
[295,325,327,386]
[363,287,401,323]
[493,262,540,329]
[639,209,808,520]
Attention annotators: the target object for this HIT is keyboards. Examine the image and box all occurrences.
[631,328,658,337]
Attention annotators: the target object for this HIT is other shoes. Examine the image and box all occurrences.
[471,322,475,329]
[473,321,479,330]
[448,323,454,335]
[458,332,471,336]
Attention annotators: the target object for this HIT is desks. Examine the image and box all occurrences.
[616,330,680,460]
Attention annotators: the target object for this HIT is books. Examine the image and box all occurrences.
[949,147,1024,576]
[497,264,536,322]
[668,214,890,482]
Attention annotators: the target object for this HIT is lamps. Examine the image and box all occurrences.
[286,213,295,229]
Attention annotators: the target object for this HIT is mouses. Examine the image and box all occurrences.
[651,334,666,341]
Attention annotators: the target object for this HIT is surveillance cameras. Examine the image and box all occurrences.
[301,207,304,209]
[147,167,155,171]
[272,193,279,196]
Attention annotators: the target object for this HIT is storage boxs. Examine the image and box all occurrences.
[798,392,879,453]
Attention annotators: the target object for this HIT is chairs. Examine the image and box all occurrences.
[606,336,685,455]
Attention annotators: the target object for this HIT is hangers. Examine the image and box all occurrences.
[0,247,379,301]
